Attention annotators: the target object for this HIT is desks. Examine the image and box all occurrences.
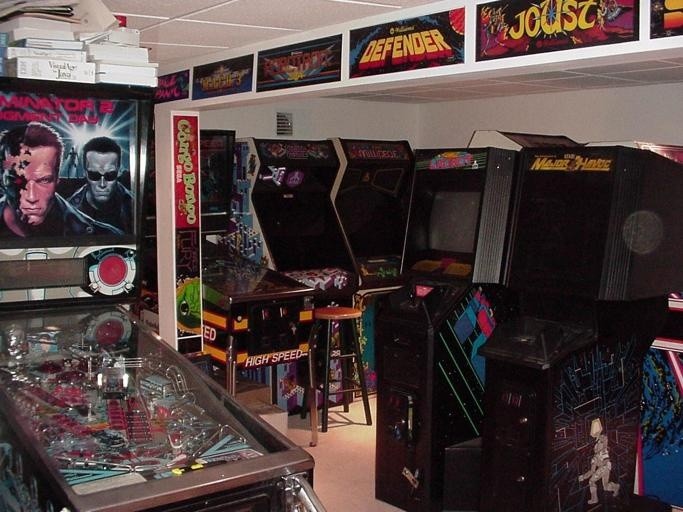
[199,246,322,447]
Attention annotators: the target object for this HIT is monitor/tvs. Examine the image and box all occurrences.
[520,206,611,301]
[341,193,406,258]
[257,196,346,269]
[425,188,482,259]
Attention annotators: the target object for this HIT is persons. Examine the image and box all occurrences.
[65,136,136,234]
[0,121,127,240]
[64,146,80,180]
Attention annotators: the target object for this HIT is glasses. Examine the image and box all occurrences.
[86,172,116,180]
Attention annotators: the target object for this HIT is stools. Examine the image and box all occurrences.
[302,306,372,433]
